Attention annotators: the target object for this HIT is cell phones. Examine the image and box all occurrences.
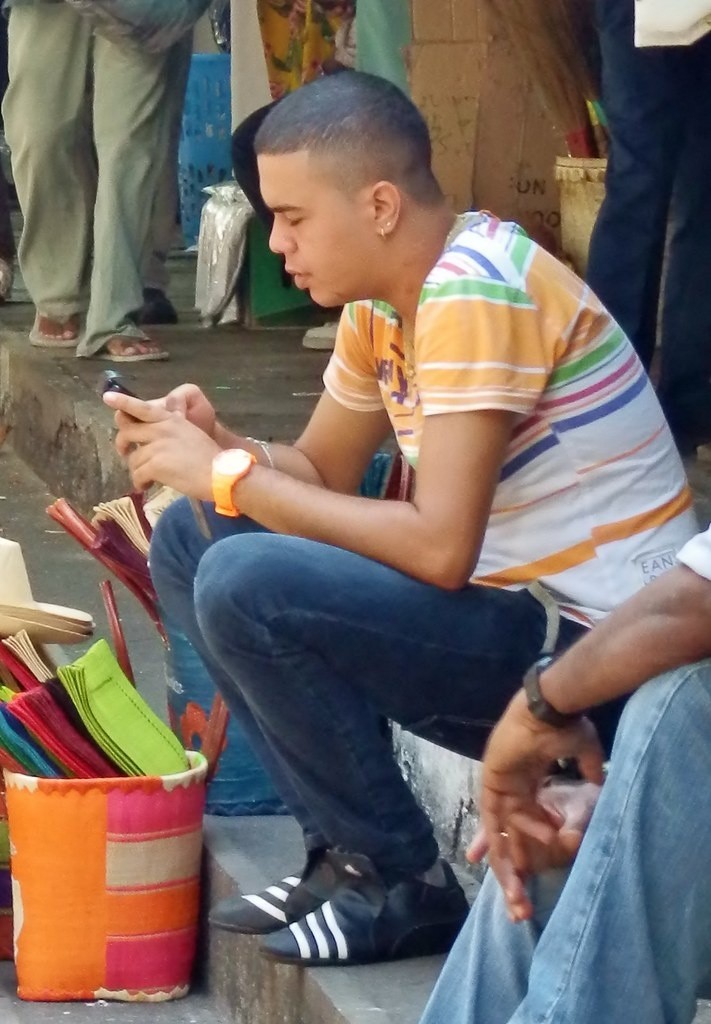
[96,370,141,402]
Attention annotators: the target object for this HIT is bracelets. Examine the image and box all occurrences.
[246,435,274,468]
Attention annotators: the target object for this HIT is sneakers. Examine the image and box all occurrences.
[207,843,331,935]
[255,849,469,965]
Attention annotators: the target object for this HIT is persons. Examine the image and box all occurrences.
[0,1,711,1024]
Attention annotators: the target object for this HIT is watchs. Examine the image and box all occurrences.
[523,655,588,728]
[210,448,260,518]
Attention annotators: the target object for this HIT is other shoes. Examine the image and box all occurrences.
[131,288,177,325]
[0,257,13,299]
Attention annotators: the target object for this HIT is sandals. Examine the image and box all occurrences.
[30,309,80,347]
[92,335,169,362]
